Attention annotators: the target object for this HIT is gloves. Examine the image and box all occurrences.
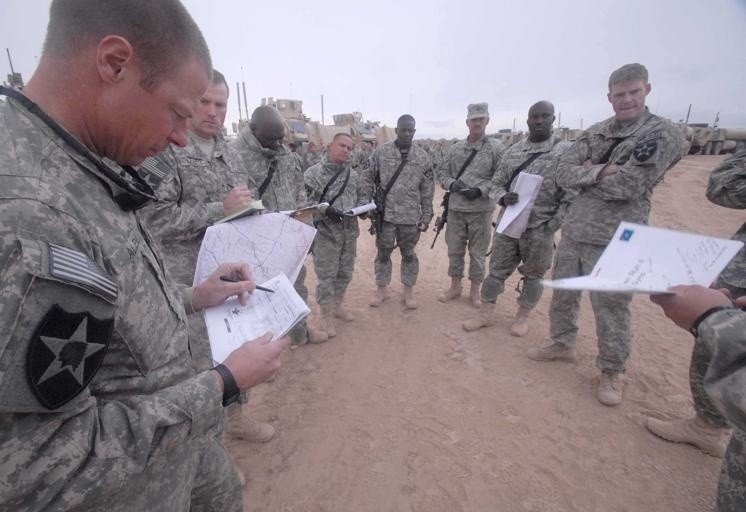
[462,188,481,200]
[326,206,344,223]
[499,191,518,205]
[451,179,466,192]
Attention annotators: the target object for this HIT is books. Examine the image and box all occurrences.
[212,199,265,226]
[202,271,312,368]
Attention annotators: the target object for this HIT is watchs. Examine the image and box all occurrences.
[208,364,240,409]
[690,305,741,339]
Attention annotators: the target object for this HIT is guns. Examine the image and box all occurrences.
[430,192,449,250]
[368,172,385,234]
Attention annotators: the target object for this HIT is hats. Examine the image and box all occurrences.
[467,103,488,120]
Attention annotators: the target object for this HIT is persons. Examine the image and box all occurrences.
[523,62,690,407]
[303,133,360,338]
[288,133,451,184]
[133,69,275,487]
[463,101,582,337]
[643,143,745,458]
[649,284,746,512]
[435,103,507,309]
[225,105,329,383]
[357,114,434,309]
[1,0,290,512]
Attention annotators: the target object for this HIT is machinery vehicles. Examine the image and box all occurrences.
[0,44,26,102]
[488,105,745,156]
[223,81,331,151]
[317,93,397,152]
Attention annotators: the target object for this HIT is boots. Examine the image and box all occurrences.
[404,284,417,308]
[469,282,482,307]
[512,305,532,336]
[225,402,274,442]
[370,286,390,305]
[463,302,495,330]
[289,297,354,349]
[438,277,462,303]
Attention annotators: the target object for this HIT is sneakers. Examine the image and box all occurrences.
[599,371,622,405]
[526,343,576,362]
[647,414,733,457]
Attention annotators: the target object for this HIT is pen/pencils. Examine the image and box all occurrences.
[219,275,274,294]
[226,182,255,200]
